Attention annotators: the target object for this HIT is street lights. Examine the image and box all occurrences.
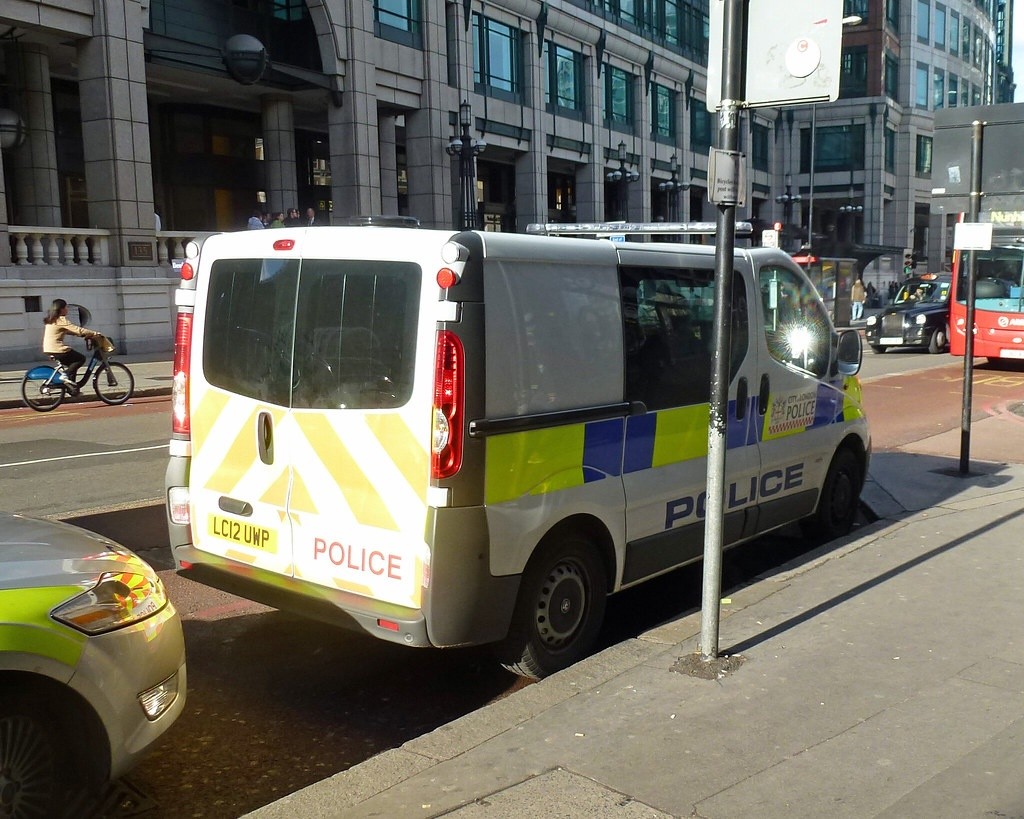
[776,171,802,254]
[804,15,865,279]
[659,153,690,242]
[839,183,864,258]
[605,139,640,222]
[445,99,487,232]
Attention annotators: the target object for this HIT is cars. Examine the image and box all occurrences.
[865,271,967,354]
[0,510,186,819]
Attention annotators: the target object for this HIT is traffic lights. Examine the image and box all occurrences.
[903,248,913,275]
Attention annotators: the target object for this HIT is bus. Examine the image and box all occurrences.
[948,213,1024,367]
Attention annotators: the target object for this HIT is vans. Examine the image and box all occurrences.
[163,221,873,683]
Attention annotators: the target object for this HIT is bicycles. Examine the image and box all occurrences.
[21,334,135,413]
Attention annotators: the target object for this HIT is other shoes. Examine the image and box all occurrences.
[59,374,78,386]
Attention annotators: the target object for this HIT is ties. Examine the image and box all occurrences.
[308,219,312,226]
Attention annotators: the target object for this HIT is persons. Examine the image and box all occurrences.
[849,281,928,321]
[247,207,324,230]
[42,298,102,396]
[155,213,161,231]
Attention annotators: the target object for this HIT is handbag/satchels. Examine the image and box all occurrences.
[102,336,115,352]
